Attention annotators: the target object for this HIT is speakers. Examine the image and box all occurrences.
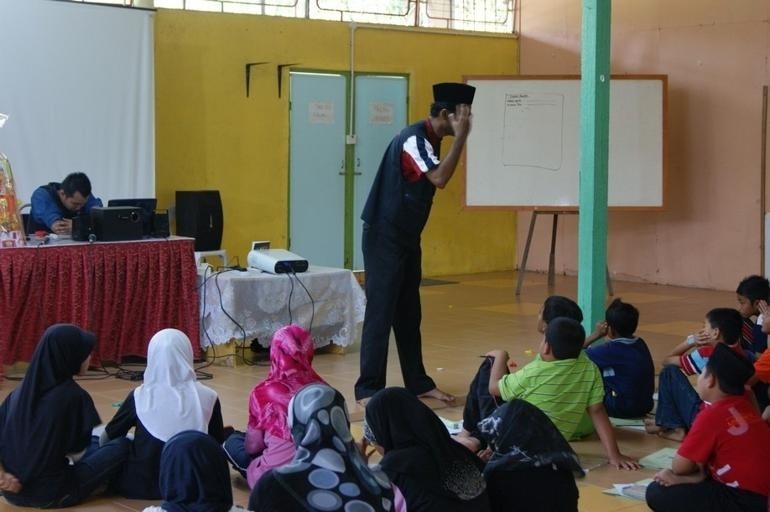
[152,210,171,238]
[91,205,142,243]
[72,216,89,239]
[175,189,224,251]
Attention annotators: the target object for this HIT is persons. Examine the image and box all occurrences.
[1,323,132,509]
[537,296,586,334]
[28,173,102,234]
[251,382,408,512]
[735,275,770,351]
[645,343,770,512]
[452,319,644,472]
[646,307,756,444]
[225,325,329,490]
[582,297,655,419]
[751,300,770,419]
[356,386,487,512]
[354,83,476,406]
[99,329,233,499]
[140,431,250,512]
[485,399,583,512]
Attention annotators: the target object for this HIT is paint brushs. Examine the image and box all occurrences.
[478,355,493,358]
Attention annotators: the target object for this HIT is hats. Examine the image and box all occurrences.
[433,82,477,105]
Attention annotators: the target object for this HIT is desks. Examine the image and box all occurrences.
[0,235,202,382]
[196,266,368,369]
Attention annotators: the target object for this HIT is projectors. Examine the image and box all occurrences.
[247,249,309,275]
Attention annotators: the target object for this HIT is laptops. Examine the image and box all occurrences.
[108,198,156,240]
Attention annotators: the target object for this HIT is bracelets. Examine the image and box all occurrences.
[688,334,695,348]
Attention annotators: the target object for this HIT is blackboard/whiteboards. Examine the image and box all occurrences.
[458,75,670,210]
